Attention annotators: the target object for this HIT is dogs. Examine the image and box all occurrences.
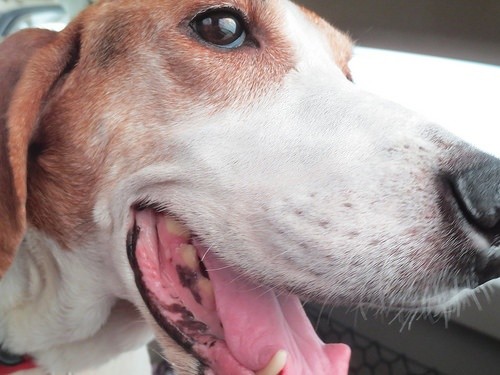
[0,0,500,375]
